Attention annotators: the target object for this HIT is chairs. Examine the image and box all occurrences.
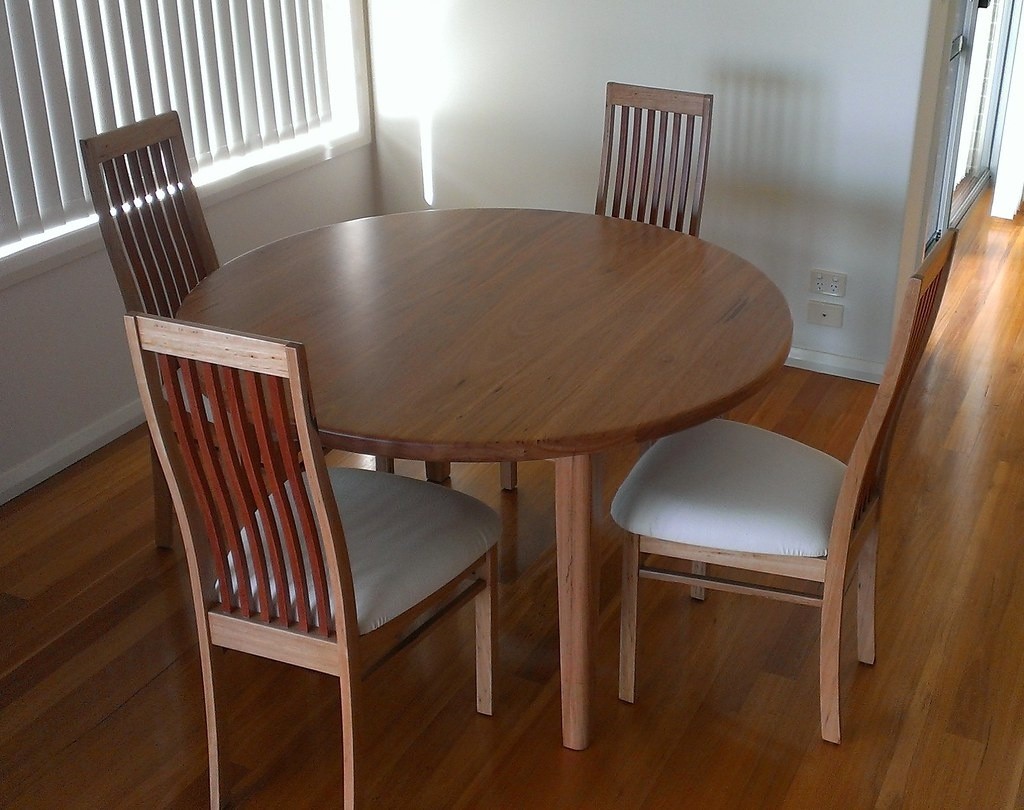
[80,110,396,551]
[117,308,498,810]
[499,79,714,488]
[615,222,962,743]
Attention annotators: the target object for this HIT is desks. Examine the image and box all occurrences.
[164,206,794,752]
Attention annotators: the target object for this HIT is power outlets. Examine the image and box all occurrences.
[809,269,847,296]
